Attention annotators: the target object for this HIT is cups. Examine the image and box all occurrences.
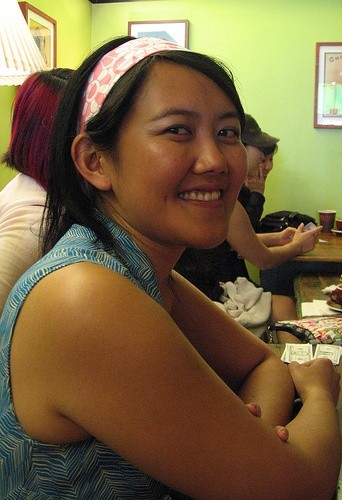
[336,219,342,231]
[318,210,337,233]
[330,284,342,306]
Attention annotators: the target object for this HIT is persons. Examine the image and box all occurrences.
[0,35,342,500]
[175,113,320,345]
[0,68,80,305]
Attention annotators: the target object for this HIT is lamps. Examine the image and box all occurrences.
[0,0,47,85]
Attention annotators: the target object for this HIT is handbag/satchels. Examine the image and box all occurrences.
[259,209,316,233]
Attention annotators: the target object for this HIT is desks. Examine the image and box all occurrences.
[289,232,342,321]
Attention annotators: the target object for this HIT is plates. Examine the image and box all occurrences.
[330,229,342,234]
[326,300,342,312]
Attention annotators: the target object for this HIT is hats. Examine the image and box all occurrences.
[240,114,280,148]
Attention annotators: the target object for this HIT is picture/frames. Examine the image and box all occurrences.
[128,21,188,49]
[313,42,342,129]
[18,2,57,70]
